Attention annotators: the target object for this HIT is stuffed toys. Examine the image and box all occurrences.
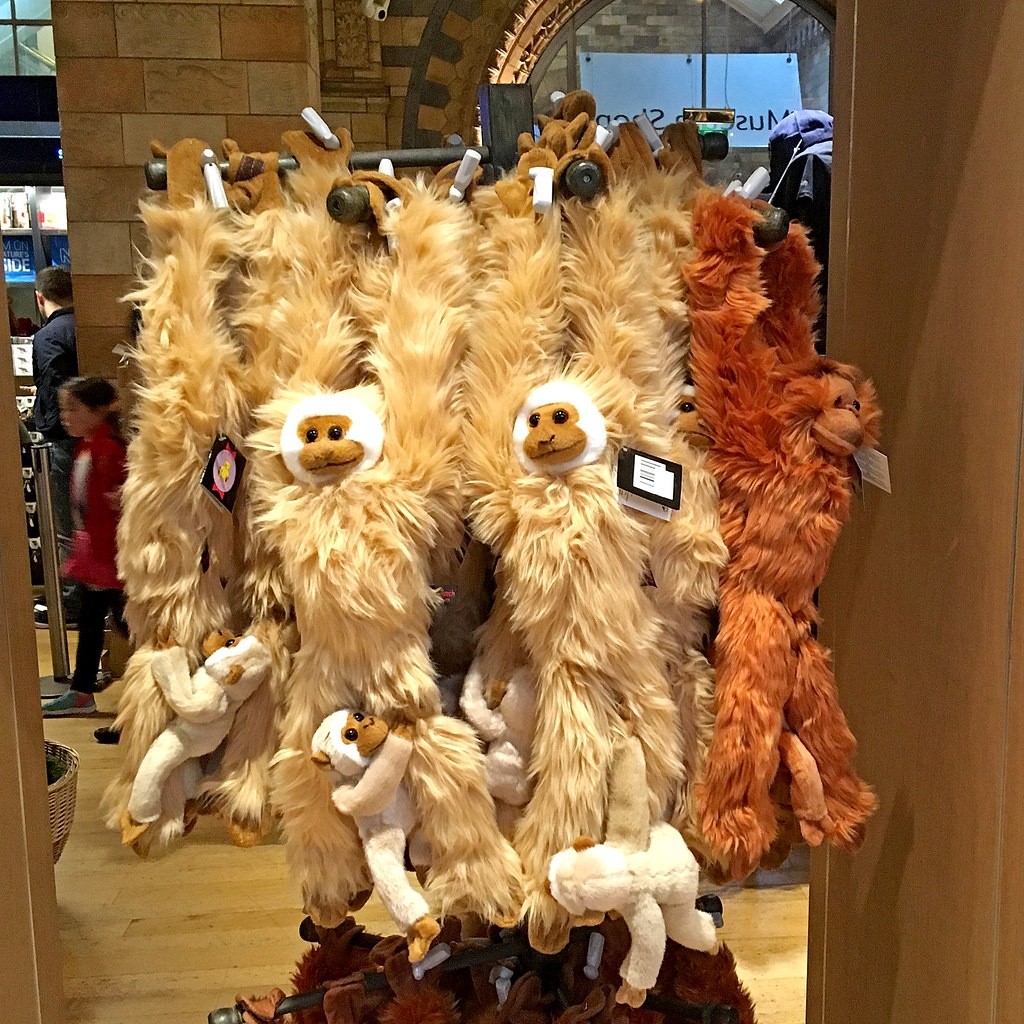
[98,92,881,1024]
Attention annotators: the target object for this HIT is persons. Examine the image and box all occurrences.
[41,372,126,717]
[33,265,83,627]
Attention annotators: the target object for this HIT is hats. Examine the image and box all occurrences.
[760,108,833,192]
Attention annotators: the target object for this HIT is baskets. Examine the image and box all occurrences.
[42,738,80,867]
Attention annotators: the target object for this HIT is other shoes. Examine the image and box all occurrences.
[94,726,119,744]
[34,596,78,628]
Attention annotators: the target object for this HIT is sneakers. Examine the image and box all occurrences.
[42,691,97,716]
